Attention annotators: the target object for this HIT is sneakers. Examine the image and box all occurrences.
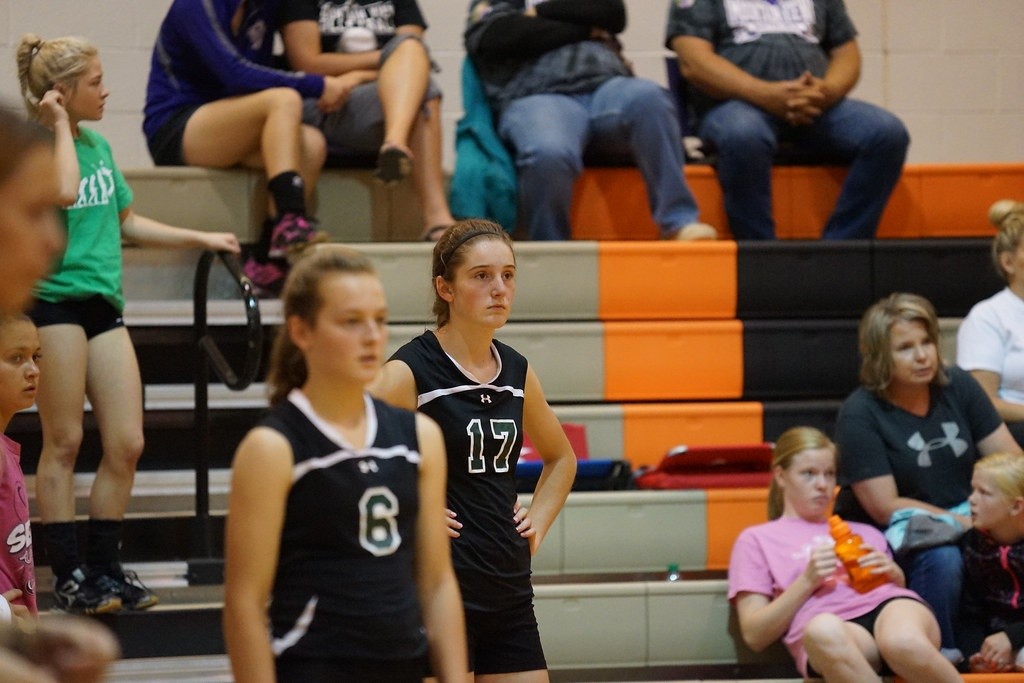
[90,569,158,611]
[53,569,122,614]
[243,251,287,300]
[268,214,327,258]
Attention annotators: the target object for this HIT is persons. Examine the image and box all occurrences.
[225,247,468,683]
[664,0,909,242]
[464,0,718,242]
[831,291,1024,675]
[959,453,1024,674]
[141,0,462,299]
[16,36,240,614]
[374,219,577,683]
[956,198,1024,452]
[0,109,121,683]
[726,425,965,683]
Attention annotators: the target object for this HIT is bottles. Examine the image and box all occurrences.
[666,564,682,581]
[827,515,891,594]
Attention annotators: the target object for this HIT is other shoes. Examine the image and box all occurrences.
[670,222,716,243]
[373,147,413,189]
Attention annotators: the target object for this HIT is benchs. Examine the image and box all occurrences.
[7,168,1024,683]
